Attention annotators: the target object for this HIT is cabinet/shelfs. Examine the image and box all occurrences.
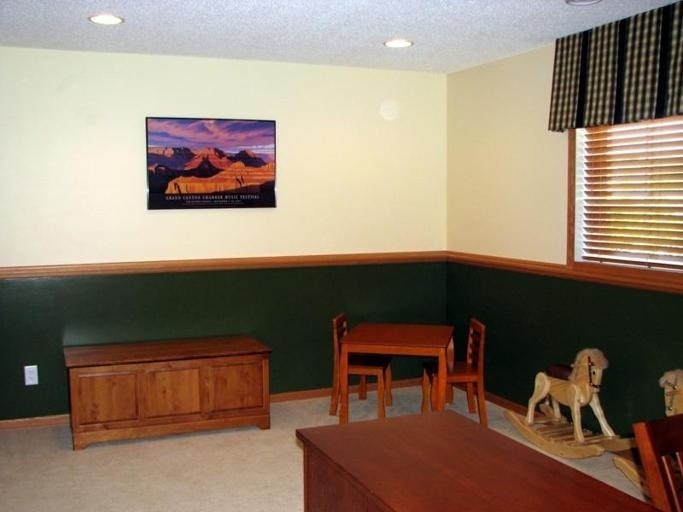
[64,334,272,451]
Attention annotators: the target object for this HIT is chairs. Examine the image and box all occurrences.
[631,412,682,512]
[329,312,392,423]
[423,318,487,427]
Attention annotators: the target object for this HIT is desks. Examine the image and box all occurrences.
[340,323,455,425]
[296,409,662,512]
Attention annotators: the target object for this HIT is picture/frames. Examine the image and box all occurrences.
[145,116,277,210]
